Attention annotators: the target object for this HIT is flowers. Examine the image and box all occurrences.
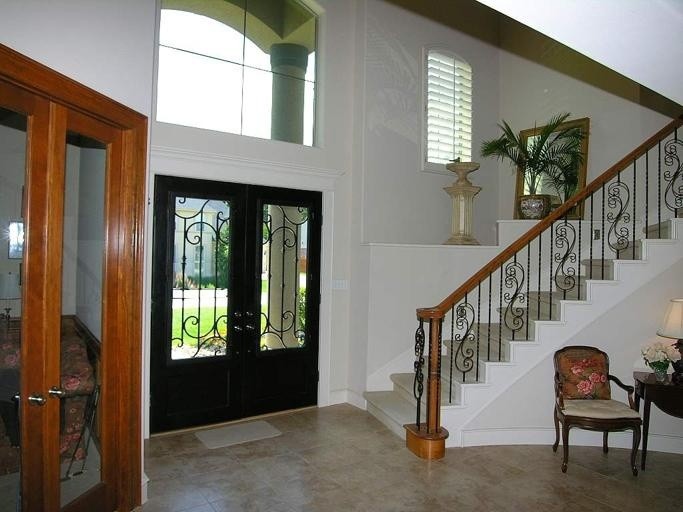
[641,342,681,371]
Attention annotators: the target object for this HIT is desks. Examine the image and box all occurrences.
[632,369,682,473]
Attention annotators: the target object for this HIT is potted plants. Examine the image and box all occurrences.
[478,112,590,221]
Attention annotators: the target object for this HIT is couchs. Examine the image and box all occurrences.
[0,315,98,477]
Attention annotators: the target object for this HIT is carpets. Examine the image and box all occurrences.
[193,420,284,450]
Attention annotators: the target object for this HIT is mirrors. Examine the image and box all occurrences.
[7,219,24,260]
[512,117,589,221]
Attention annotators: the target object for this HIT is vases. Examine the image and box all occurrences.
[654,369,666,382]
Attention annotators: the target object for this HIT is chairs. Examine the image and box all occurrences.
[551,343,641,476]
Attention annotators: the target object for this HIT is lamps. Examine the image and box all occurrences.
[655,299,683,379]
[0,271,23,336]
